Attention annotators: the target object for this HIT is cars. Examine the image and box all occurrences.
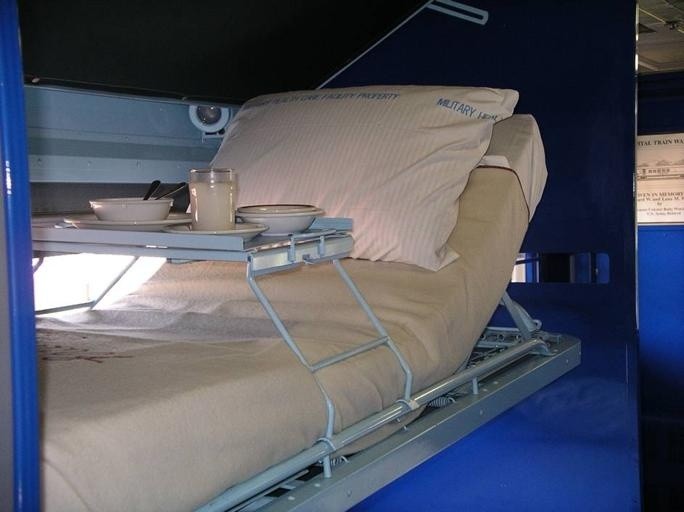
[190,168,235,233]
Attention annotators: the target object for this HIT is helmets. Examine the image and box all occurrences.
[62,213,268,243]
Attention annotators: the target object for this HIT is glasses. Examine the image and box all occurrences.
[188,98,235,142]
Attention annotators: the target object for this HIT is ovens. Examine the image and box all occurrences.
[29,112,581,512]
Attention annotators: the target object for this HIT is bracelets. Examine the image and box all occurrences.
[233,205,324,236]
[89,197,174,221]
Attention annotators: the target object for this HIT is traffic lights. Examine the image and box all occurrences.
[187,85,518,272]
